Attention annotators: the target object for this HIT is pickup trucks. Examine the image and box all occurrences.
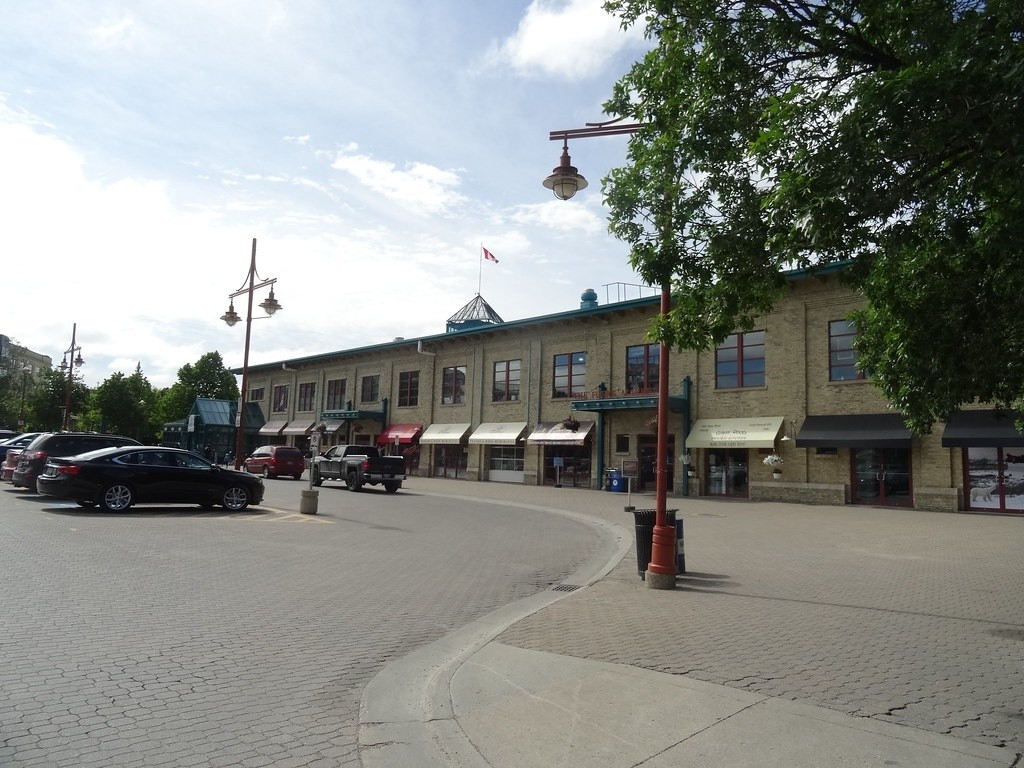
[310,444,408,494]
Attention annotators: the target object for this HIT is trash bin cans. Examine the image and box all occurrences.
[606,469,623,491]
[631,508,686,582]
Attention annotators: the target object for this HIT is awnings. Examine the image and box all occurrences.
[419,423,471,444]
[796,413,921,449]
[310,419,346,435]
[685,416,785,448]
[528,420,595,446]
[942,409,1024,447]
[469,422,527,445]
[258,421,288,436]
[282,420,316,435]
[377,424,423,443]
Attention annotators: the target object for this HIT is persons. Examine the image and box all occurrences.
[204,443,211,459]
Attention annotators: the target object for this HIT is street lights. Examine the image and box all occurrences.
[57,323,86,432]
[219,238,284,471]
[540,116,690,590]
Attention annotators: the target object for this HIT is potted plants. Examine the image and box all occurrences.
[772,468,782,479]
[687,466,696,476]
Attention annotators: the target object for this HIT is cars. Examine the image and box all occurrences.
[0,448,25,481]
[242,445,306,480]
[0,430,20,442]
[36,446,265,513]
[0,431,46,466]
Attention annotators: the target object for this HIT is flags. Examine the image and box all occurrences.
[483,247,499,263]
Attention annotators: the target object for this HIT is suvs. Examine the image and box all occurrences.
[12,433,163,491]
